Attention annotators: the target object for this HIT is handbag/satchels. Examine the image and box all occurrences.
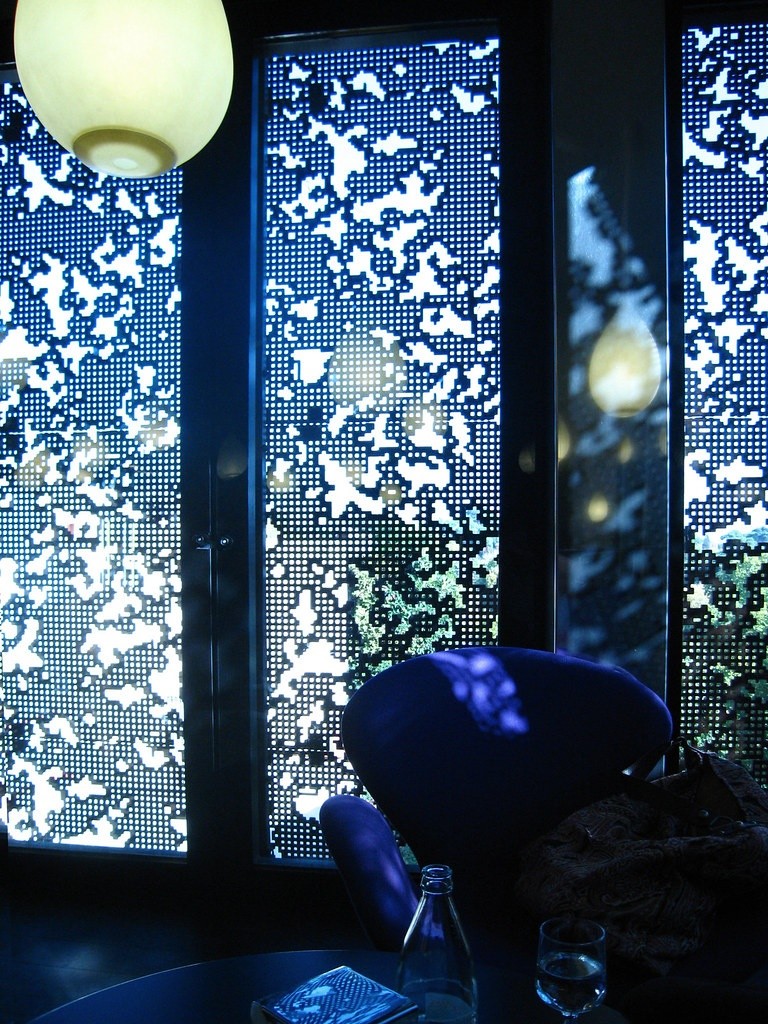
[510,734,768,1024]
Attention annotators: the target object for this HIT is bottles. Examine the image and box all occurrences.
[393,863,478,1024]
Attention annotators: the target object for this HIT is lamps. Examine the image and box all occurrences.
[589,301,660,417]
[13,0,234,176]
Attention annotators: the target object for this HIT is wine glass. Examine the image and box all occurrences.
[535,918,607,1024]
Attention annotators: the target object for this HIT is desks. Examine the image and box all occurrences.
[24,950,627,1024]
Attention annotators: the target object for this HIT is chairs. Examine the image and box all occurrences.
[320,646,672,966]
